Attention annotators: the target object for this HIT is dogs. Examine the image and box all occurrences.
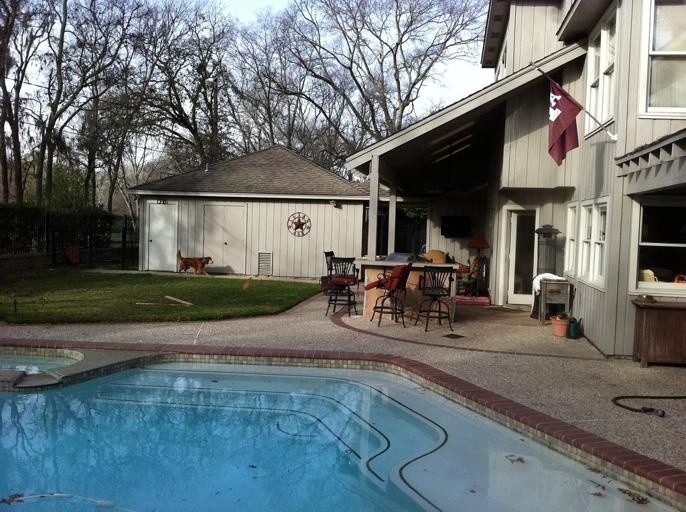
[177,250,214,277]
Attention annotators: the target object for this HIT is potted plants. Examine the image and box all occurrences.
[549,311,569,337]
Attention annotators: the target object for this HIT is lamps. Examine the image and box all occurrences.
[468,232,489,298]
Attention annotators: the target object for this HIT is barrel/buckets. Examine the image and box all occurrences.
[549,316,569,337]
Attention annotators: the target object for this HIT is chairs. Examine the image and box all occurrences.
[321,251,486,333]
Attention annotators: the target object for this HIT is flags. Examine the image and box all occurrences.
[538,69,585,166]
